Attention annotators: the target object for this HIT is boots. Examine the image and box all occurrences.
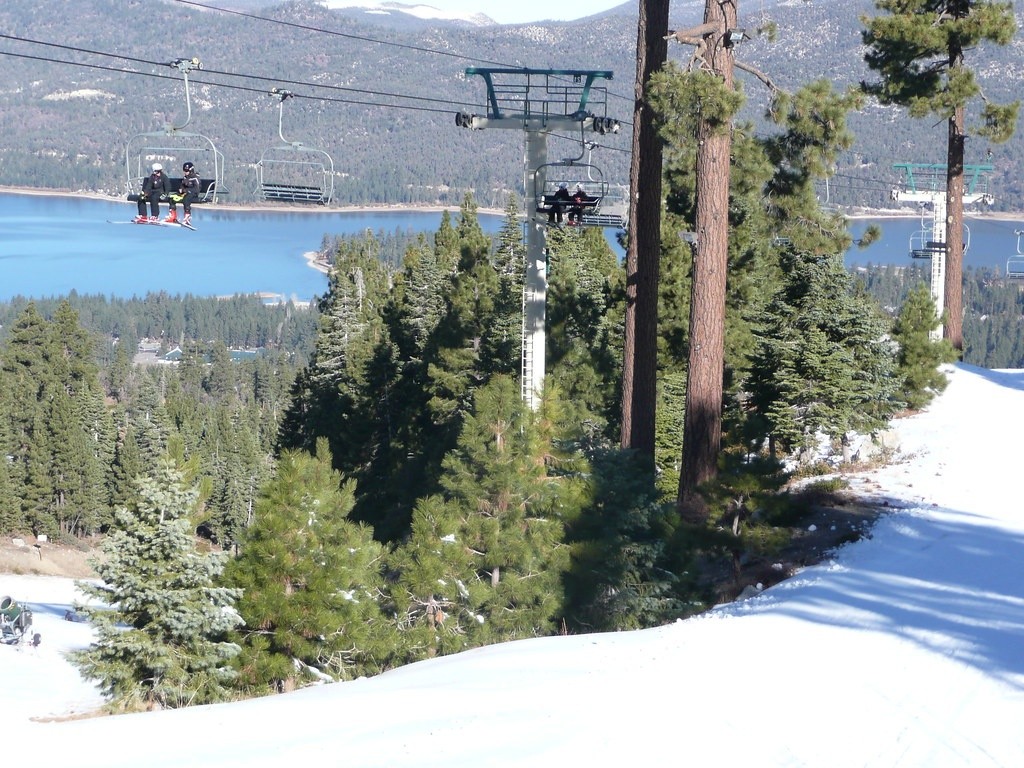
[184,213,191,225]
[163,209,177,222]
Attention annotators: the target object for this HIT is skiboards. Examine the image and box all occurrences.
[136,214,198,232]
[105,218,167,227]
[532,217,565,234]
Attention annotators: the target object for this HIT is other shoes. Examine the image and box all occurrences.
[557,220,564,225]
[546,220,555,225]
[568,219,573,226]
[576,221,583,227]
[135,214,147,221]
[149,215,159,222]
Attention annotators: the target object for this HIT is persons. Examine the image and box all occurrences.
[132,162,170,223]
[547,182,569,223]
[161,162,201,225]
[568,185,587,226]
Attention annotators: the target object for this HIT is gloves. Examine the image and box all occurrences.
[187,188,193,195]
[575,197,582,205]
[177,188,184,195]
[556,198,562,204]
[140,190,149,200]
[160,193,167,200]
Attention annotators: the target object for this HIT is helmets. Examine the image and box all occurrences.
[576,183,583,191]
[151,163,163,174]
[182,162,194,174]
[559,182,567,189]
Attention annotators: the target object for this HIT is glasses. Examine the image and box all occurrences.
[559,186,563,188]
[155,170,163,174]
[183,169,189,172]
